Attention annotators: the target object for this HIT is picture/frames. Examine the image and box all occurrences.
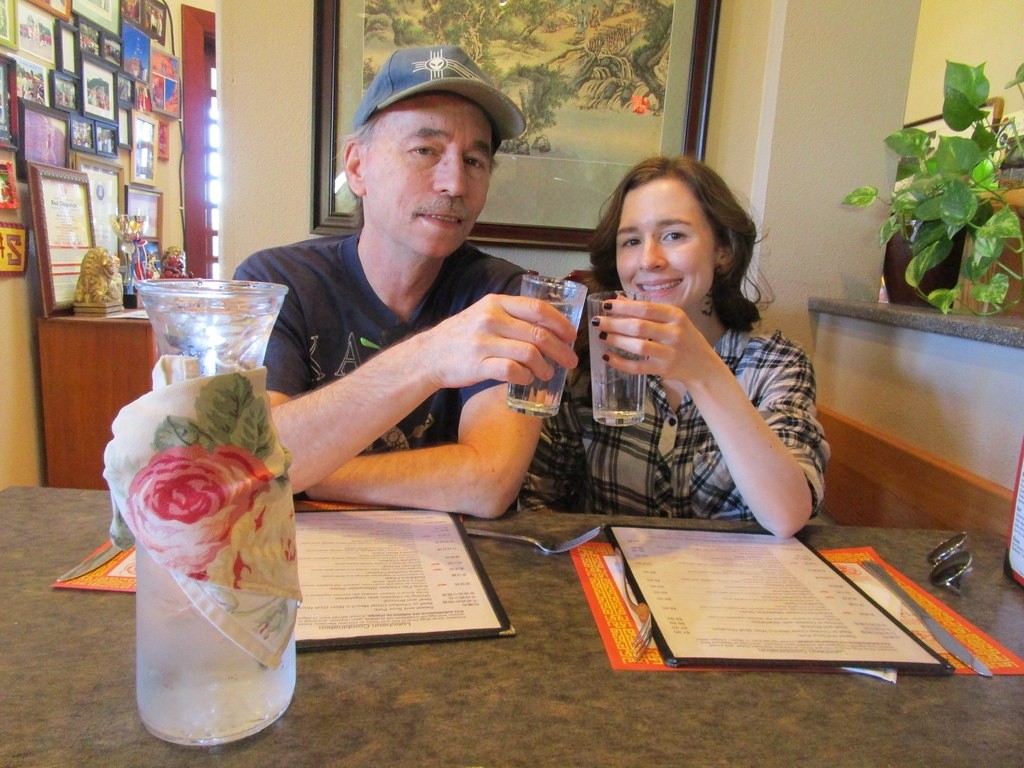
[120,0,145,31]
[124,185,164,240]
[150,46,180,118]
[124,240,162,294]
[136,81,152,116]
[50,69,82,115]
[0,0,18,51]
[309,0,723,257]
[54,18,83,80]
[68,111,96,155]
[121,19,151,85]
[77,16,104,59]
[102,28,125,71]
[81,52,119,128]
[118,101,132,151]
[130,110,158,187]
[25,159,95,319]
[18,96,71,177]
[119,72,138,109]
[27,0,72,22]
[94,120,119,159]
[147,0,166,46]
[0,163,21,209]
[74,153,126,266]
[156,116,170,161]
[0,53,20,152]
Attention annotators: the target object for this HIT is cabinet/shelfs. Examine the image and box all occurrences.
[34,306,160,492]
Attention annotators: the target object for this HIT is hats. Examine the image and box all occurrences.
[351,45,526,156]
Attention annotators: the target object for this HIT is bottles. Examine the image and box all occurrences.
[131,278,293,749]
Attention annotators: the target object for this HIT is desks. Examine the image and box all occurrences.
[0,483,1024,768]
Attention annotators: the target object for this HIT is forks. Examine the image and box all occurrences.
[614,547,653,661]
[466,526,600,553]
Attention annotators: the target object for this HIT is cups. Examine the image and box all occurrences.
[507,275,587,417]
[587,291,652,427]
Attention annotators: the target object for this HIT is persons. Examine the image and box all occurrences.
[234,44,578,520]
[521,152,832,540]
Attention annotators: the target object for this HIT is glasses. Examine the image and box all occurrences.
[928,532,973,595]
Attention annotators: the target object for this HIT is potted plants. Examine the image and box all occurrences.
[840,61,1024,318]
